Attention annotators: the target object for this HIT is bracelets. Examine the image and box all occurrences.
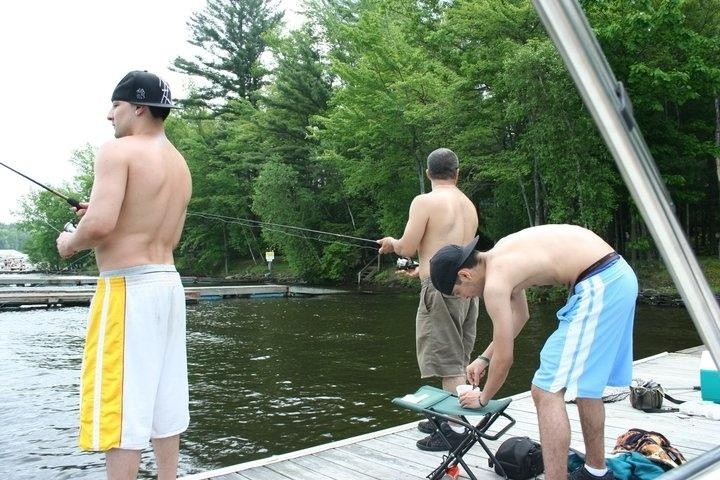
[478,355,490,363]
[478,394,488,407]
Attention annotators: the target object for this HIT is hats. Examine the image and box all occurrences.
[429,234,481,296]
[111,69,182,109]
[426,148,459,169]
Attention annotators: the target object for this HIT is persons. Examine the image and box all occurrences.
[430,224,639,479]
[375,148,479,453]
[55,68,192,480]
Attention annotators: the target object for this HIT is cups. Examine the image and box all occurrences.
[456,385,481,407]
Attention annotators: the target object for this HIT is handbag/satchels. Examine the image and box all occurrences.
[494,436,545,480]
[628,377,666,411]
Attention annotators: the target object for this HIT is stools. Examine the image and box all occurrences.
[392,385,516,479]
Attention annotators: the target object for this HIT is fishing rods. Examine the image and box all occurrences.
[0,162,85,214]
[186,211,419,271]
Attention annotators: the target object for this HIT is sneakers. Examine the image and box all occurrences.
[416,417,473,451]
[568,465,613,480]
